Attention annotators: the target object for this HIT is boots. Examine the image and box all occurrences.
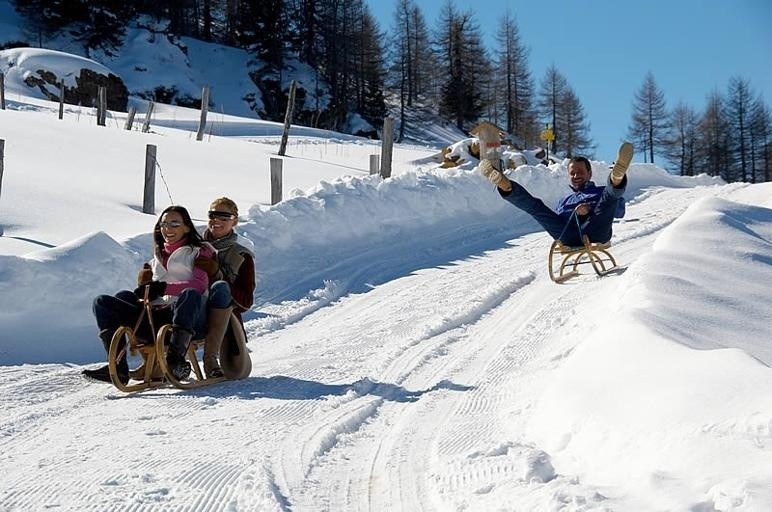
[478,159,511,192]
[81,325,131,389]
[610,143,635,186]
[202,305,234,379]
[164,326,197,383]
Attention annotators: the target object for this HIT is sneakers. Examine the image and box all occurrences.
[128,363,146,381]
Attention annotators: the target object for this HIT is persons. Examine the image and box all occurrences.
[478,141,636,250]
[81,203,219,383]
[127,197,256,378]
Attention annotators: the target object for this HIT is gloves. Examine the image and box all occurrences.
[136,263,154,284]
[134,279,167,299]
[194,254,220,276]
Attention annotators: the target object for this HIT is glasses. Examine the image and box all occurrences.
[208,211,234,221]
[158,222,183,229]
